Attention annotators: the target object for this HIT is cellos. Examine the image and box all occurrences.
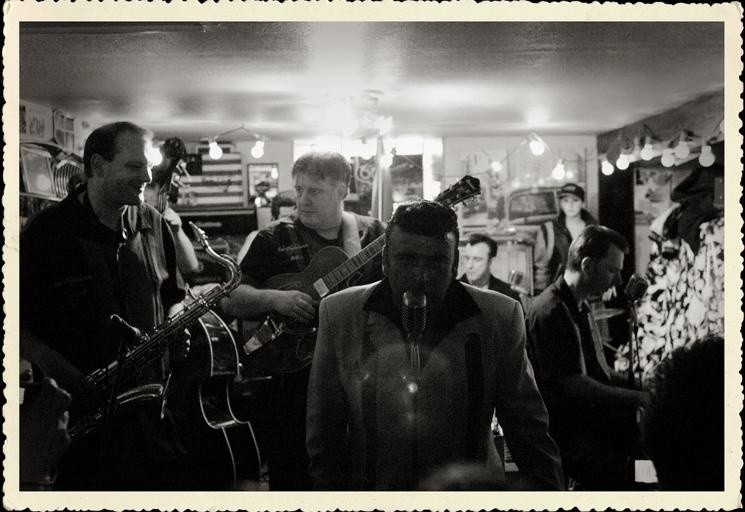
[154,136,261,491]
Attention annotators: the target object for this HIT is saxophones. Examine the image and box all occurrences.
[66,221,242,438]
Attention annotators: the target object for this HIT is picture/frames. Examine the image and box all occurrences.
[19,145,57,197]
[246,162,280,204]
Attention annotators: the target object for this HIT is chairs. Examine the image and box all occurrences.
[491,414,577,491]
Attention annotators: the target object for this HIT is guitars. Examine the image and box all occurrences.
[238,174,481,374]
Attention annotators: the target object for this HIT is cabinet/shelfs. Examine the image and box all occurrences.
[166,201,259,284]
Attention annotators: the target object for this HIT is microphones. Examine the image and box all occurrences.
[402,290,428,336]
[508,269,523,285]
[625,274,648,304]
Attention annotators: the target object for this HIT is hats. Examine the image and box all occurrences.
[557,183,584,202]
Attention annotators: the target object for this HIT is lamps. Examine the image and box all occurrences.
[147,124,442,197]
[489,120,715,180]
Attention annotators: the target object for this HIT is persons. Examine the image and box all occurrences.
[19,121,724,491]
